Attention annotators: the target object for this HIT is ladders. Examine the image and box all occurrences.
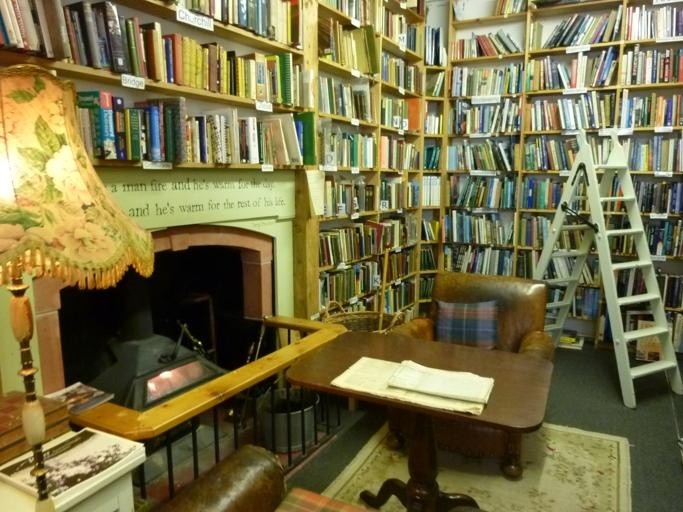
[530,129,682,408]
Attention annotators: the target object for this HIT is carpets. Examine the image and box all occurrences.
[319,412,633,512]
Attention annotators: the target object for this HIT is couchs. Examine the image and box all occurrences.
[382,270,555,478]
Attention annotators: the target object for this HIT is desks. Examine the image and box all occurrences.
[286,330,553,512]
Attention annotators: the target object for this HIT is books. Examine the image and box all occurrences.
[0,426,147,505]
[43,380,114,416]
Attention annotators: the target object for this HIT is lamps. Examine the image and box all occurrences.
[0,61,154,511]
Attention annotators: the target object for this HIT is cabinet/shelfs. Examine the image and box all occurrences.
[0,0,683,357]
